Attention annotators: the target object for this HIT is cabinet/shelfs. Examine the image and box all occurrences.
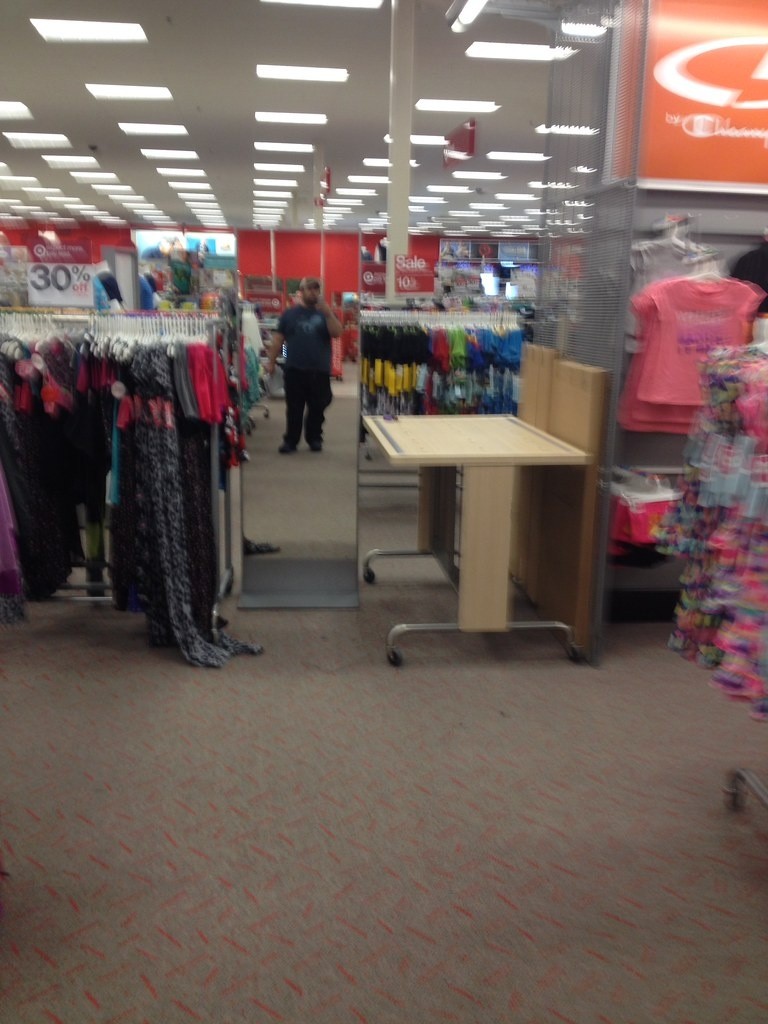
[438,235,550,275]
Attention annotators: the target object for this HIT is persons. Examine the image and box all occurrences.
[264,277,342,454]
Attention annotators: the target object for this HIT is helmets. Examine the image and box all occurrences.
[194,242,209,251]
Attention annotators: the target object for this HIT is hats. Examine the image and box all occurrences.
[175,234,192,255]
[299,276,319,289]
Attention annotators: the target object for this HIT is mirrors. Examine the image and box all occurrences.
[234,228,362,609]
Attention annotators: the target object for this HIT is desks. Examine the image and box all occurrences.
[362,415,593,670]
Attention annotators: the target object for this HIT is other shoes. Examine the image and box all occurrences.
[310,440,323,452]
[278,441,297,454]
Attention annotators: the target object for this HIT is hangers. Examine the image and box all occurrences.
[611,214,768,510]
[0,298,253,361]
[354,307,520,331]
